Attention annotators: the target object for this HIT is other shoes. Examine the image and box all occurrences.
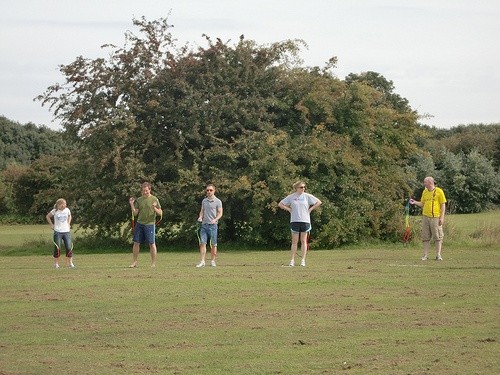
[129,264,136,268]
[211,261,216,267]
[55,264,59,269]
[69,262,75,268]
[289,260,294,266]
[421,256,429,261]
[196,261,205,267]
[300,260,305,267]
[436,256,442,261]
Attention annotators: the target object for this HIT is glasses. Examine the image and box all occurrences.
[296,186,305,189]
[207,189,213,192]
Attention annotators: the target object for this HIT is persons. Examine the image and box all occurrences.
[278,181,322,266]
[128,182,163,268]
[46,198,74,269]
[195,184,223,268]
[410,176,447,261]
